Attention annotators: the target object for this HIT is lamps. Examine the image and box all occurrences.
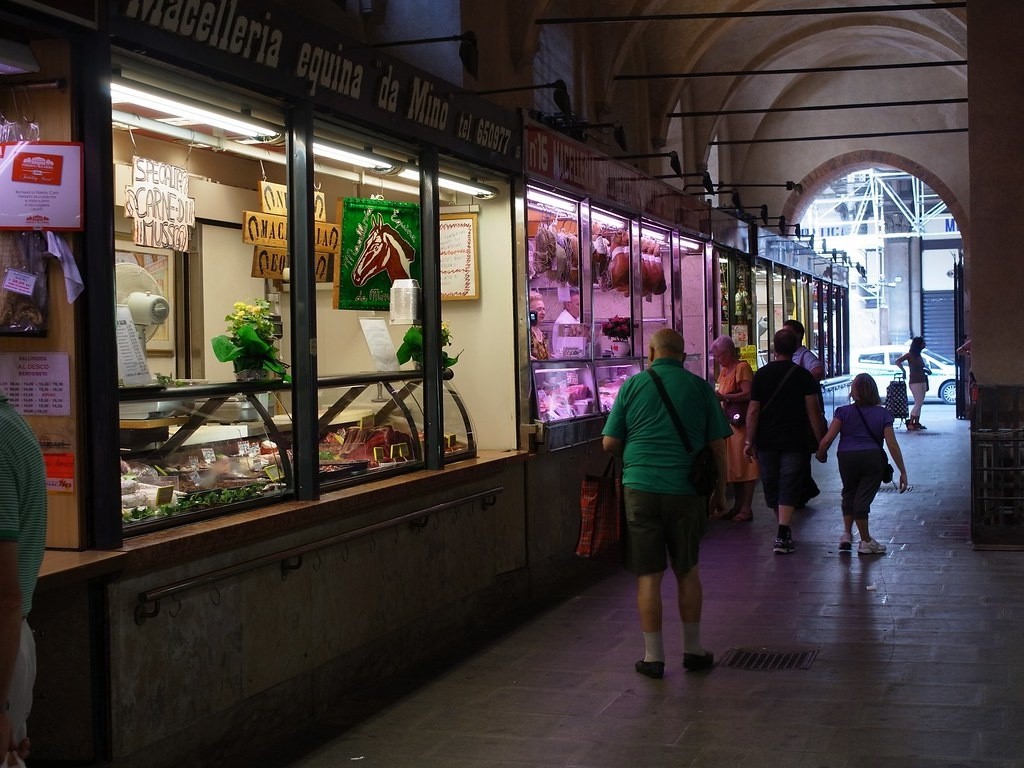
[110,74,500,201]
[369,31,868,279]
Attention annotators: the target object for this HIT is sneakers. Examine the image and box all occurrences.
[839,534,854,550]
[858,538,886,554]
[774,530,795,554]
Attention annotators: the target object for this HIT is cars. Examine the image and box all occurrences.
[855,344,958,406]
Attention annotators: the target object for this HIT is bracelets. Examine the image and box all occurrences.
[745,441,752,444]
[0,701,10,713]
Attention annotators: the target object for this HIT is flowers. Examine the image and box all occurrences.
[218,299,276,349]
[409,324,451,351]
[601,316,634,343]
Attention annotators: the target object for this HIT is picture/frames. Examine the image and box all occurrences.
[437,214,480,301]
[114,230,178,358]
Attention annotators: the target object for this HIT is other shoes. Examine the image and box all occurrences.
[909,423,915,430]
[733,511,752,523]
[636,658,665,680]
[914,423,926,429]
[724,507,739,518]
[683,651,713,669]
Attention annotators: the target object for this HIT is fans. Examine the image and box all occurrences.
[116,264,169,384]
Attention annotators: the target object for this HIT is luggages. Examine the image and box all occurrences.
[885,373,910,427]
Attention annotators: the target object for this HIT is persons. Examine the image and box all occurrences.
[956,339,972,357]
[743,329,823,554]
[784,320,825,509]
[552,289,580,359]
[896,337,932,430]
[707,335,760,523]
[530,290,548,360]
[0,387,48,768]
[601,328,735,679]
[816,373,908,554]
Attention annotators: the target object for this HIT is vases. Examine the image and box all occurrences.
[227,336,278,379]
[609,339,631,357]
[407,345,447,370]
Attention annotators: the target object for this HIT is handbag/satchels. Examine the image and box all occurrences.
[882,450,892,483]
[723,360,753,427]
[694,447,719,489]
[574,449,628,558]
